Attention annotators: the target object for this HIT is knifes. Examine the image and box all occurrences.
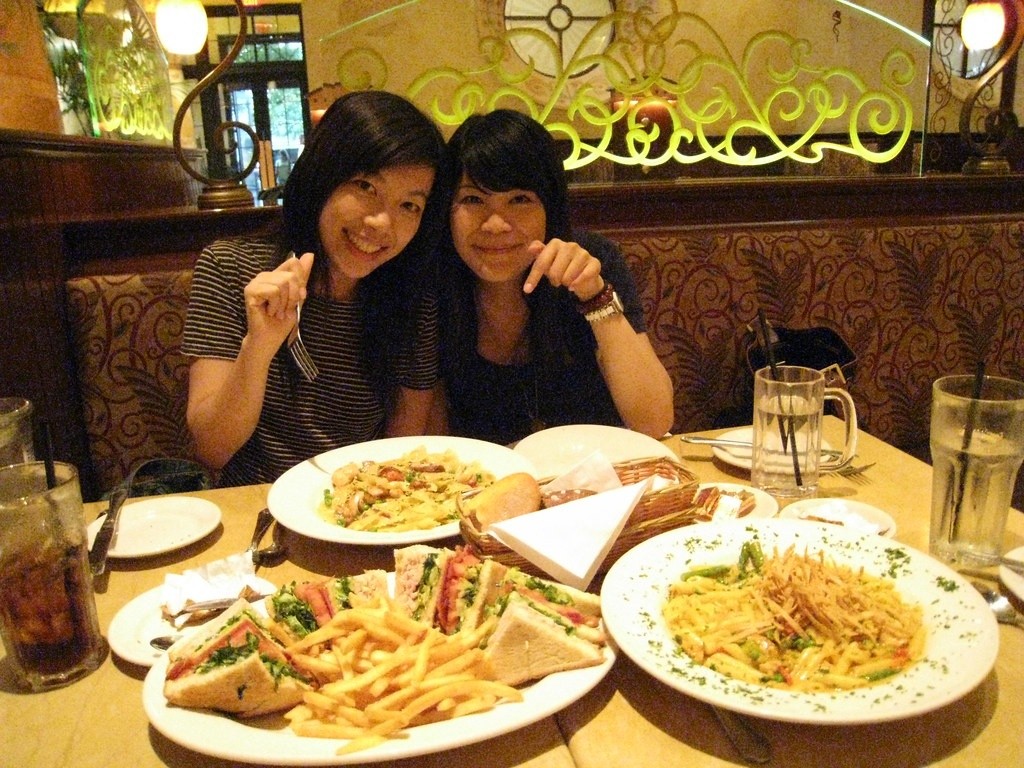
[679,435,860,459]
[89,486,129,579]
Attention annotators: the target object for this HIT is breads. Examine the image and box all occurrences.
[466,472,596,526]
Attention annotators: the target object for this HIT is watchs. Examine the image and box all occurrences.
[584,291,624,321]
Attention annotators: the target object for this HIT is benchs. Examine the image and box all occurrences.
[66,179,1024,500]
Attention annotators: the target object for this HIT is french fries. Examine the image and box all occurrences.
[291,591,522,757]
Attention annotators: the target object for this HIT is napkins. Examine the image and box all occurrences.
[488,476,680,592]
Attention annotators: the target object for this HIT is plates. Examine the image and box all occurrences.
[85,496,222,559]
[265,438,540,546]
[712,427,831,474]
[599,518,1001,726]
[511,424,680,492]
[779,497,897,540]
[106,555,273,667]
[997,546,1024,603]
[141,572,618,768]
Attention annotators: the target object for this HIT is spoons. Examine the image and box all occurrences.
[971,582,1024,629]
[260,522,285,559]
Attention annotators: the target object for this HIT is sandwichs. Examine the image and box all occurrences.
[163,544,605,717]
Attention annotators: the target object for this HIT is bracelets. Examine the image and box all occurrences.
[575,279,614,315]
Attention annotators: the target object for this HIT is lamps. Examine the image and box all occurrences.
[958,0,1024,173]
[156,0,262,208]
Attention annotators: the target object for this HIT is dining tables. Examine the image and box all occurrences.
[0,416,1024,768]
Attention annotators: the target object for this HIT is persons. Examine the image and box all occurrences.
[179,90,673,486]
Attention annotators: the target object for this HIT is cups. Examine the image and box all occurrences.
[0,460,110,694]
[0,396,36,466]
[749,364,858,500]
[928,373,1024,569]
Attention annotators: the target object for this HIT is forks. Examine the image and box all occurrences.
[836,461,877,478]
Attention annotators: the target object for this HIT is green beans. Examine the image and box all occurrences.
[680,541,765,581]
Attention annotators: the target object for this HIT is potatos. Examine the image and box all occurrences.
[665,574,923,688]
